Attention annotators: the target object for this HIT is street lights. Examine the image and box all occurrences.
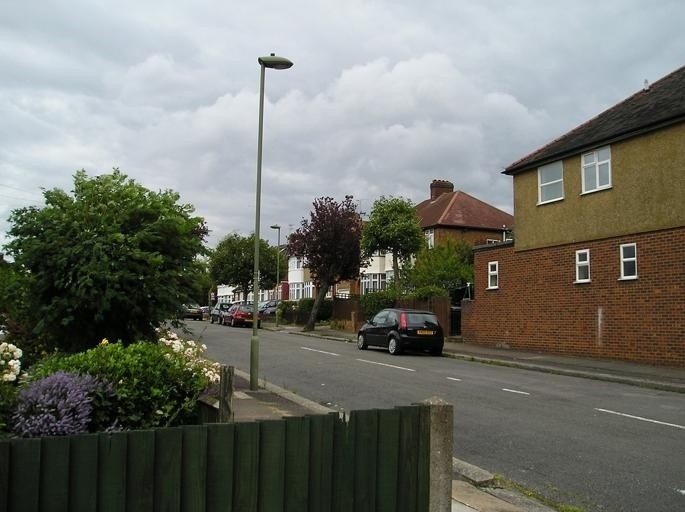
[270,224,281,327]
[250,53,294,391]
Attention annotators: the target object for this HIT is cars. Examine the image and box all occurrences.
[181,301,276,327]
[357,307,444,355]
